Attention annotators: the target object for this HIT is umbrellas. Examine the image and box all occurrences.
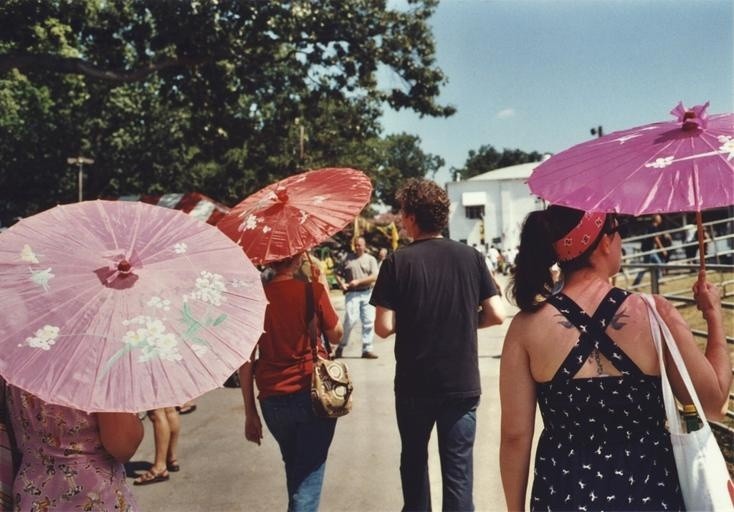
[213,165,373,267]
[523,100,734,273]
[0,198,270,416]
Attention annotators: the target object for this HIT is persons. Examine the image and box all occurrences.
[470,240,518,280]
[632,214,673,294]
[366,176,505,511]
[131,406,181,488]
[238,252,343,512]
[497,188,731,512]
[334,236,380,361]
[681,218,711,274]
[0,373,144,511]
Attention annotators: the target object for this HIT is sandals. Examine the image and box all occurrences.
[133,456,179,485]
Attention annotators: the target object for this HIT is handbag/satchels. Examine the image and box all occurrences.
[667,410,734,512]
[311,359,353,419]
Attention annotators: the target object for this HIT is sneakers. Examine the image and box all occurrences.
[335,348,344,357]
[362,351,377,358]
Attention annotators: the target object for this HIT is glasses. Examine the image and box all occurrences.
[607,214,637,236]
